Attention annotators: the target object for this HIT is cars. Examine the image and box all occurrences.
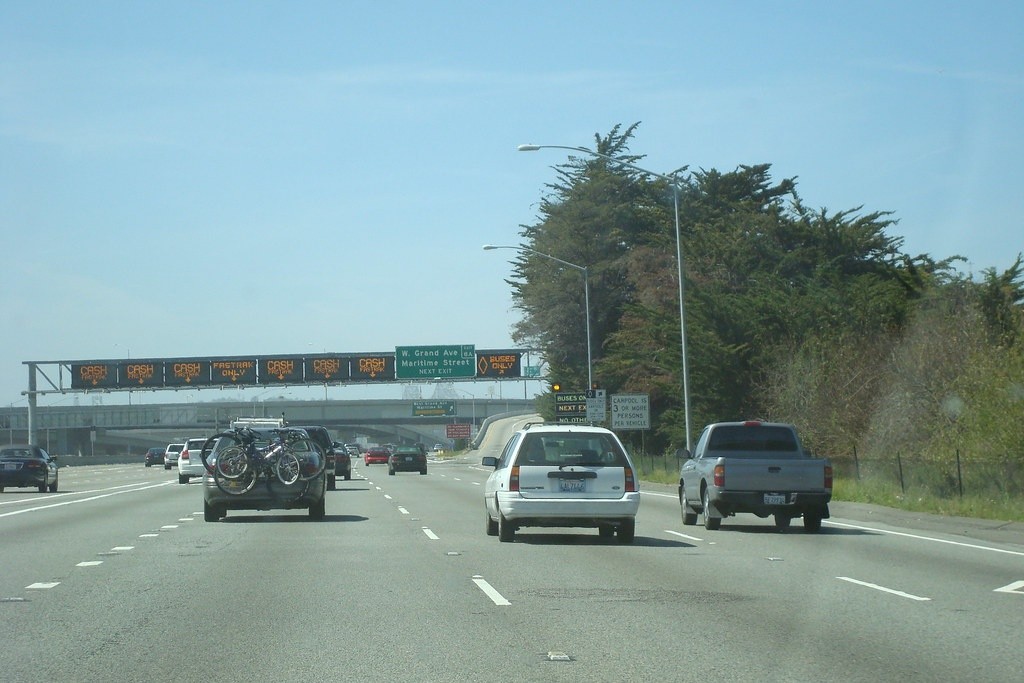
[331,441,360,482]
[163,444,186,471]
[364,443,398,467]
[387,445,429,475]
[423,445,430,453]
[144,447,166,467]
[0,444,60,493]
[434,443,444,452]
[175,439,221,484]
[201,427,335,525]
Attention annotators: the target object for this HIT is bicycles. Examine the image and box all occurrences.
[200,411,329,496]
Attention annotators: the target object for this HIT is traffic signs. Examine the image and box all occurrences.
[412,399,458,416]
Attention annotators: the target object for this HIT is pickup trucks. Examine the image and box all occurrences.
[295,423,340,491]
[672,420,835,534]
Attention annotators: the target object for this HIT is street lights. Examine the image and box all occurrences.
[518,143,694,463]
[482,244,595,426]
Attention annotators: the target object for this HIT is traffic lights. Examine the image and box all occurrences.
[552,382,561,393]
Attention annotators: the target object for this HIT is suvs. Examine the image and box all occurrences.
[482,420,641,541]
